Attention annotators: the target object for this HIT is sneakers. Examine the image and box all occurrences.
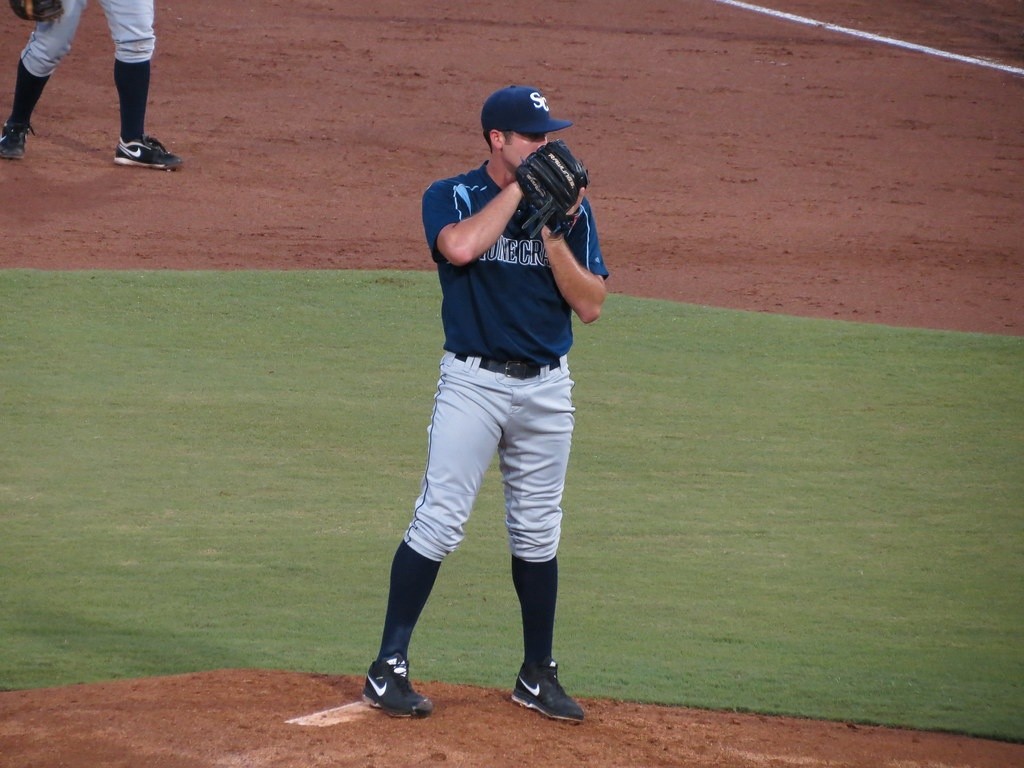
[360,653,434,719]
[510,657,585,722]
[113,134,184,170]
[0,115,36,159]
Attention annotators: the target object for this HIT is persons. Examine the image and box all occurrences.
[0,0,183,171]
[361,85,609,722]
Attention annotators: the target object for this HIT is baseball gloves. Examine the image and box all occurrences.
[9,0,65,21]
[514,138,590,239]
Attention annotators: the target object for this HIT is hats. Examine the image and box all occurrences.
[481,83,573,134]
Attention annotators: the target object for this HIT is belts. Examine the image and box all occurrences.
[453,353,561,379]
